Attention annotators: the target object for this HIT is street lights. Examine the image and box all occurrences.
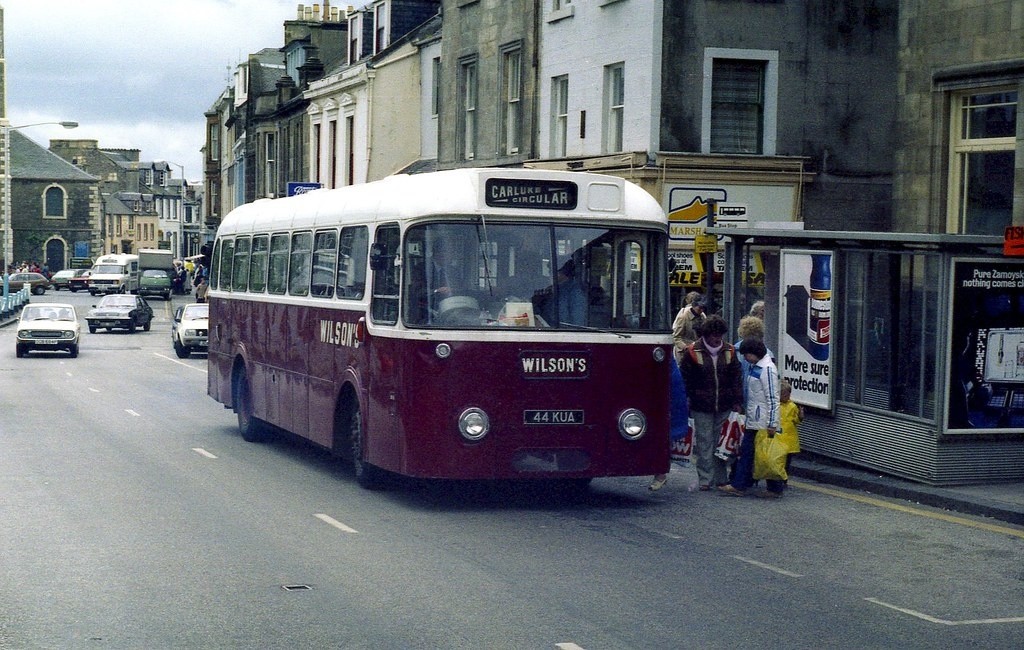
[154,160,184,258]
[2,121,79,320]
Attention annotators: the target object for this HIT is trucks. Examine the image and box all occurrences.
[87,252,140,296]
[137,247,174,301]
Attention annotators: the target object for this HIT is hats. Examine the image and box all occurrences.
[547,255,576,271]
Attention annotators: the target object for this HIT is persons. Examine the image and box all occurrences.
[734,316,775,410]
[680,314,744,491]
[649,356,688,491]
[1,261,49,277]
[410,236,463,324]
[780,381,804,489]
[750,301,764,319]
[718,337,781,498]
[171,258,209,302]
[674,291,707,366]
[546,256,587,326]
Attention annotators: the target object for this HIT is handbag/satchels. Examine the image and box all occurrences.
[753,429,788,480]
[670,417,695,468]
[714,411,746,461]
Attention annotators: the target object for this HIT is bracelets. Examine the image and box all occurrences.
[434,289,437,292]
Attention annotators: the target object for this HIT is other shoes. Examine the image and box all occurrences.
[715,483,726,488]
[648,474,667,491]
[699,485,709,490]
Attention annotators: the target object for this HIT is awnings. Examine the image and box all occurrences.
[186,255,204,260]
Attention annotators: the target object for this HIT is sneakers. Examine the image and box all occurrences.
[718,484,744,496]
[754,490,783,498]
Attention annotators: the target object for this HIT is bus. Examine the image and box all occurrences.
[203,164,675,499]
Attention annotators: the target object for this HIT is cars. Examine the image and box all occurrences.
[0,272,51,296]
[84,292,154,333]
[50,268,91,293]
[14,303,81,358]
[171,302,210,358]
